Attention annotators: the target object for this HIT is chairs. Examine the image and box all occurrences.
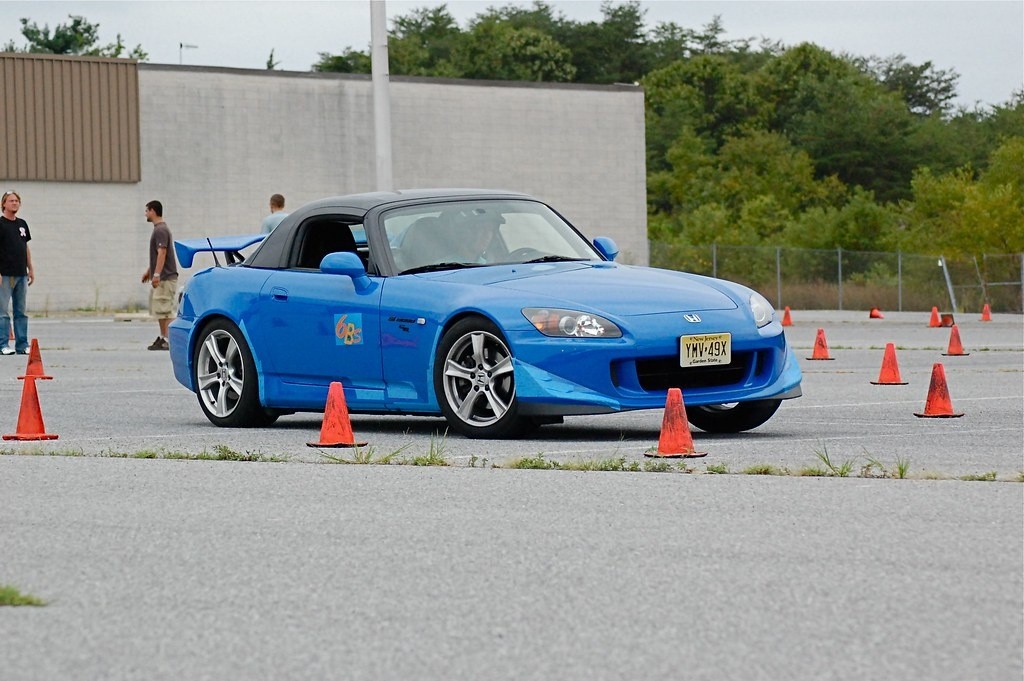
[388,216,455,274]
[301,220,361,275]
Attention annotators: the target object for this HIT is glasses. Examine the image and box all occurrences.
[2,190,19,200]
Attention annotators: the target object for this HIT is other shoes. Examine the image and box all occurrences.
[1,347,15,355]
[17,346,30,354]
[148,336,169,350]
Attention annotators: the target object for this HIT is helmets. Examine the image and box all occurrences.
[440,203,506,247]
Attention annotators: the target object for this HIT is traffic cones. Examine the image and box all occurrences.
[940,325,970,357]
[915,362,966,418]
[12,337,54,382]
[305,381,368,450]
[869,307,885,319]
[3,379,57,443]
[980,303,993,322]
[780,306,794,327]
[939,313,956,326]
[641,386,706,459]
[926,306,942,327]
[869,342,911,386]
[805,327,836,361]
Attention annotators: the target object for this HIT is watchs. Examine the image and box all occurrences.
[153,273,160,277]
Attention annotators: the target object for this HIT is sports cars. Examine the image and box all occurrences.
[167,188,804,436]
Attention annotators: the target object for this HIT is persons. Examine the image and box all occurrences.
[0,190,34,355]
[433,205,505,265]
[262,194,288,236]
[141,200,179,350]
